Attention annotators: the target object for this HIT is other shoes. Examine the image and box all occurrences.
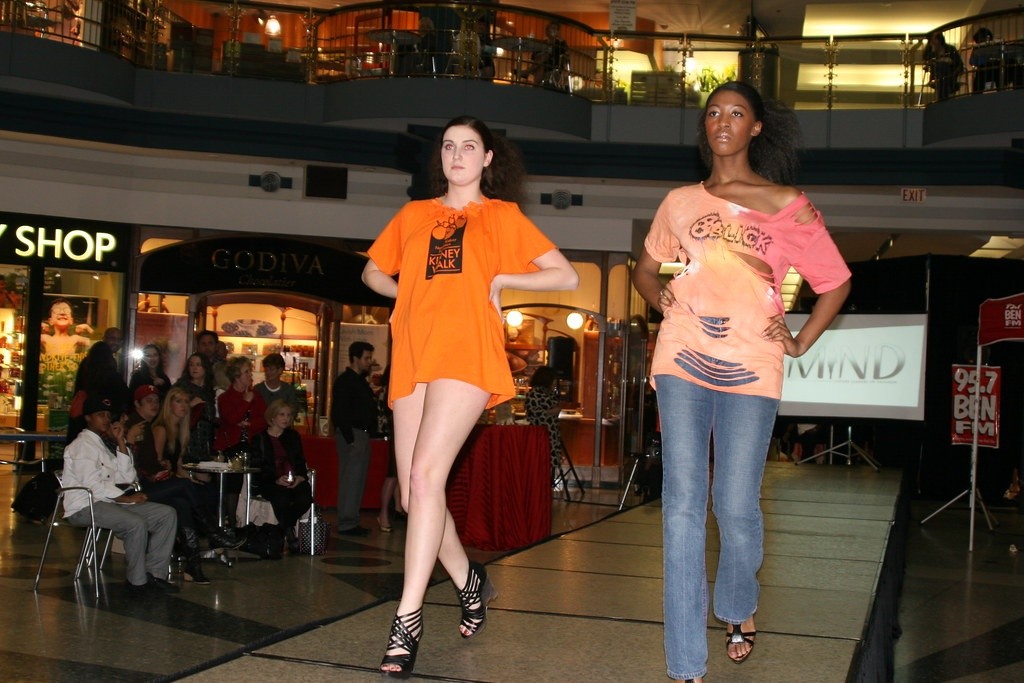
[125,583,145,600]
[287,539,300,554]
[339,525,372,537]
[148,576,180,594]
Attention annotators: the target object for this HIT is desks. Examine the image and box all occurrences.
[182,462,261,566]
[488,36,552,84]
[972,43,1024,89]
[364,28,419,78]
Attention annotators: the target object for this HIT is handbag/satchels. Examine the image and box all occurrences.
[10,472,61,524]
[216,443,252,466]
[298,519,331,554]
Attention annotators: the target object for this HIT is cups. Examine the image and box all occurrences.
[133,425,144,442]
[217,455,225,462]
[286,474,295,486]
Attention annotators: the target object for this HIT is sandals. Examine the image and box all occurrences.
[674,678,704,683]
[725,623,757,664]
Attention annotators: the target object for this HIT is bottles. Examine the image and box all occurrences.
[227,451,248,469]
[227,342,317,426]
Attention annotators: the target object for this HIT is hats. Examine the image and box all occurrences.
[83,396,113,415]
[133,384,160,400]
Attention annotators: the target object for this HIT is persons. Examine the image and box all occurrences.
[59,328,312,588]
[377,361,404,532]
[473,20,496,79]
[512,21,567,79]
[525,366,563,464]
[630,81,853,677]
[361,114,578,673]
[332,342,380,535]
[968,28,1005,93]
[417,17,434,70]
[925,32,962,100]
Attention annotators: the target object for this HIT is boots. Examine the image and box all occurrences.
[198,509,244,550]
[180,527,211,584]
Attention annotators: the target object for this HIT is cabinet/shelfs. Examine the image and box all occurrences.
[102,0,149,64]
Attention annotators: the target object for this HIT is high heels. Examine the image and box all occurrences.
[453,559,498,640]
[379,605,424,679]
[377,517,393,533]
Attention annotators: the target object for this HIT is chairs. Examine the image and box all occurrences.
[33,458,315,598]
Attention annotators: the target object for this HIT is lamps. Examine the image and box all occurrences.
[264,15,280,36]
